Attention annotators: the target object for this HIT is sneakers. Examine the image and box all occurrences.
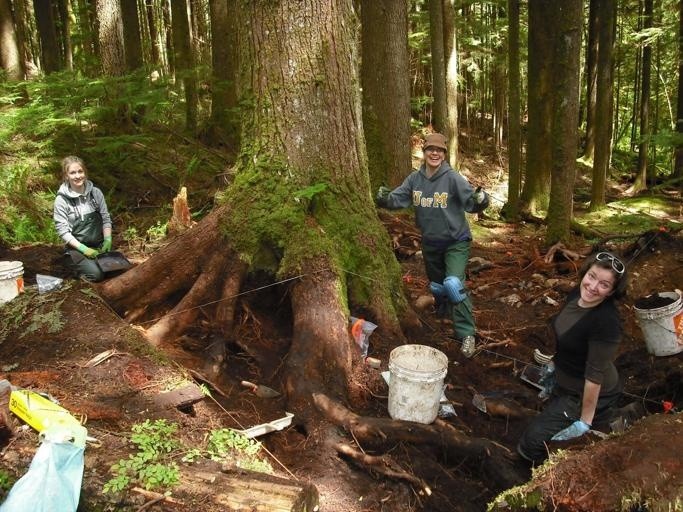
[460,335,476,358]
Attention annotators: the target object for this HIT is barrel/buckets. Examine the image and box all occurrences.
[631,287,683,357]
[0,260,25,308]
[386,344,448,424]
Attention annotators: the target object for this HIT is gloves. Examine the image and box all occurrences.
[77,244,98,258]
[376,186,392,206]
[101,236,112,253]
[551,420,593,441]
[472,185,485,205]
[539,362,556,384]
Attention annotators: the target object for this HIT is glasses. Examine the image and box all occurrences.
[425,147,444,153]
[590,252,626,281]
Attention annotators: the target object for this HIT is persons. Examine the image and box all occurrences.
[52,155,114,282]
[514,249,628,467]
[374,132,492,357]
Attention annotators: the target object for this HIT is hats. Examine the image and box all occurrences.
[423,133,449,152]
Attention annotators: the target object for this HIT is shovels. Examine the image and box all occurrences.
[95,239,131,272]
[468,385,487,415]
[242,380,281,399]
